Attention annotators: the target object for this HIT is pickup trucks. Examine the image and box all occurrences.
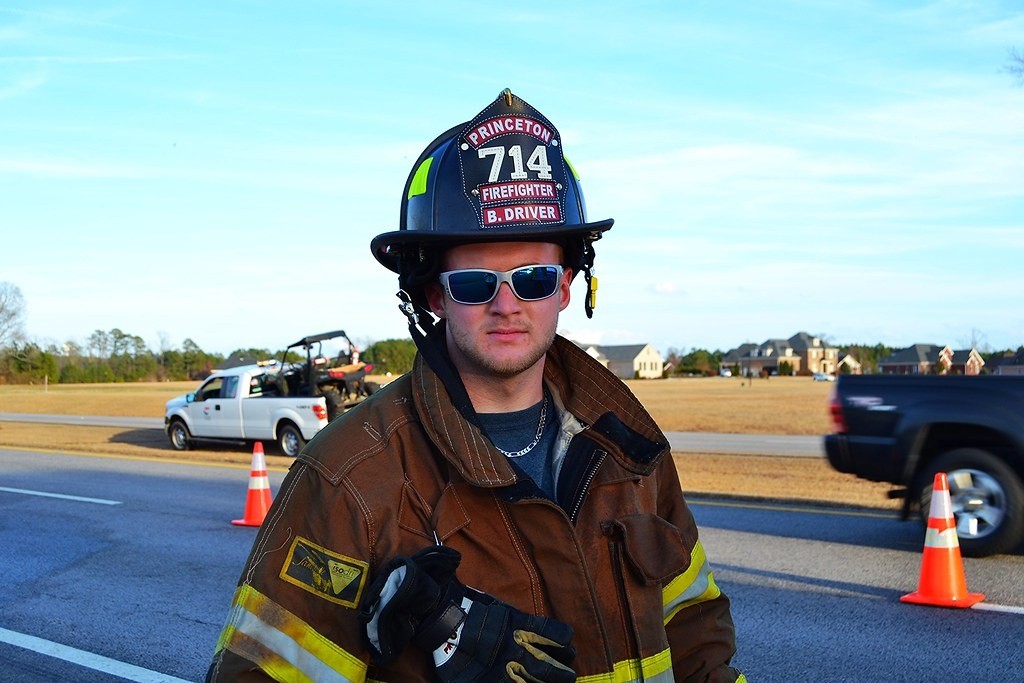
[824,373,1024,557]
[164,329,328,456]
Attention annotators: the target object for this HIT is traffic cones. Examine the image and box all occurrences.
[901,472,986,608]
[347,347,361,386]
[231,442,273,525]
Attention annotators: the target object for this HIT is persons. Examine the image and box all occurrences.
[206,89,746,683]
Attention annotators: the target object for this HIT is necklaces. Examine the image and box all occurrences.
[496,390,547,458]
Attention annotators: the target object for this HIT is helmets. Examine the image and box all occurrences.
[370,91,613,275]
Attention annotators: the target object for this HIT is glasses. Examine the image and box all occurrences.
[431,265,564,305]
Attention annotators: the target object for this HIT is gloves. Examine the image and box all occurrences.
[359,547,576,683]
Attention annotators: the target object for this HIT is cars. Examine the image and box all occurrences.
[814,372,836,382]
